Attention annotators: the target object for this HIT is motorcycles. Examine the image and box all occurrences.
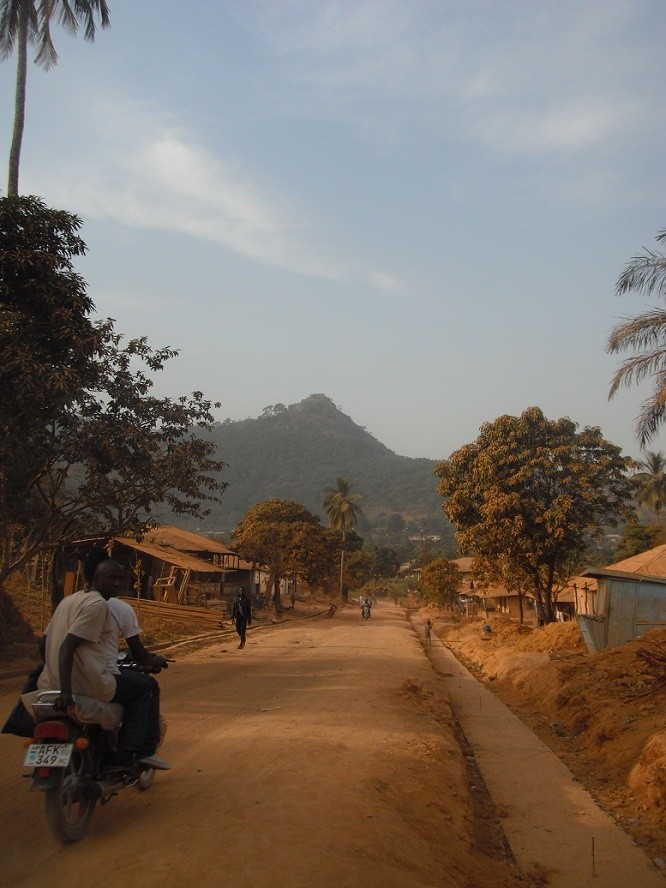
[23,649,176,845]
[361,606,370,621]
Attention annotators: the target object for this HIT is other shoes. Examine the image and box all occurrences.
[120,763,139,787]
[237,638,246,649]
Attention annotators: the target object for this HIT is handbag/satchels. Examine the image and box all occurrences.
[1,664,43,738]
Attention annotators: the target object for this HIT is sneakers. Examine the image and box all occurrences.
[137,753,171,769]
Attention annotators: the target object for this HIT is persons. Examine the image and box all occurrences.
[231,586,252,649]
[359,594,372,618]
[483,624,491,633]
[39,561,171,771]
[424,620,434,646]
